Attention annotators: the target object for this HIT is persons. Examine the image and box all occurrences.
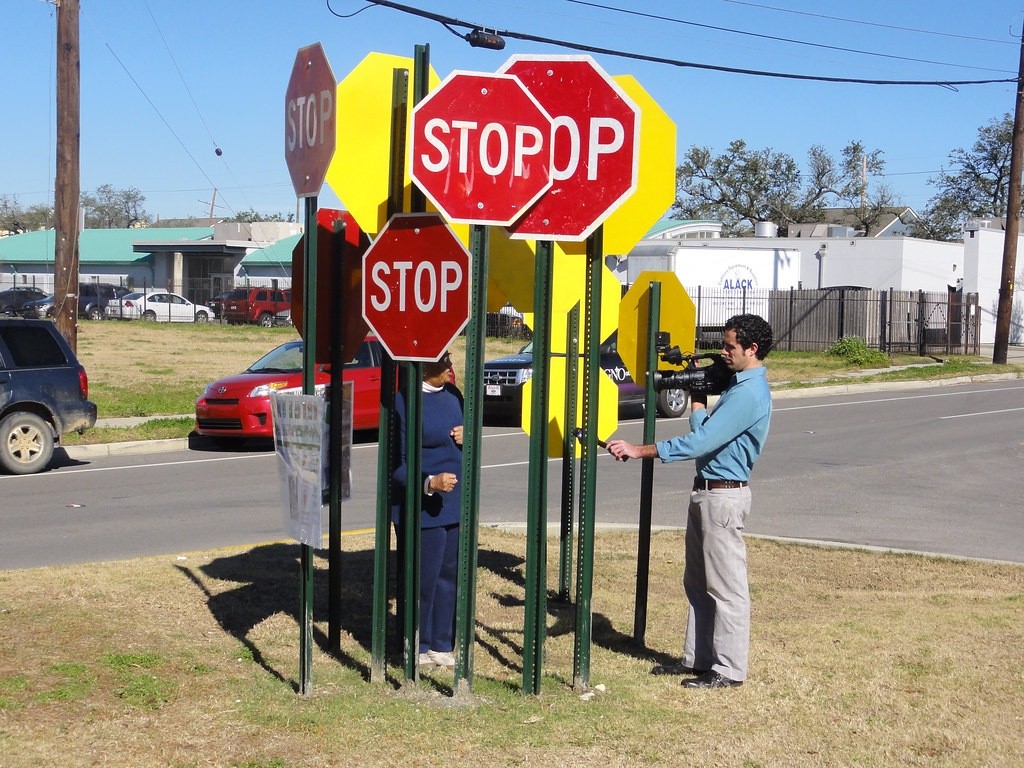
[606,315,772,688]
[389,349,466,668]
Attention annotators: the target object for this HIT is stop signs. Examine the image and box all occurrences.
[284,41,338,199]
[362,212,474,363]
[408,69,556,227]
[491,54,638,244]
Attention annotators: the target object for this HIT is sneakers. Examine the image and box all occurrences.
[681,669,743,689]
[652,662,712,676]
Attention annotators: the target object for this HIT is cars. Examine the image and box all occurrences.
[3,286,53,297]
[192,336,456,441]
[0,317,98,475]
[205,291,233,317]
[103,290,215,323]
[0,291,48,314]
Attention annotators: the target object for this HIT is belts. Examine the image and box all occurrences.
[693,477,748,490]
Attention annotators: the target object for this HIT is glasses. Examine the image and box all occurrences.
[441,353,452,363]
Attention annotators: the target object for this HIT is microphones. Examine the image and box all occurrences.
[572,427,628,462]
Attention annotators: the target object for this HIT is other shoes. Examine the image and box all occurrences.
[418,653,437,670]
[428,650,455,667]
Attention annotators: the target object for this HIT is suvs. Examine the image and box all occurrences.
[483,337,690,428]
[21,283,132,320]
[221,286,292,328]
